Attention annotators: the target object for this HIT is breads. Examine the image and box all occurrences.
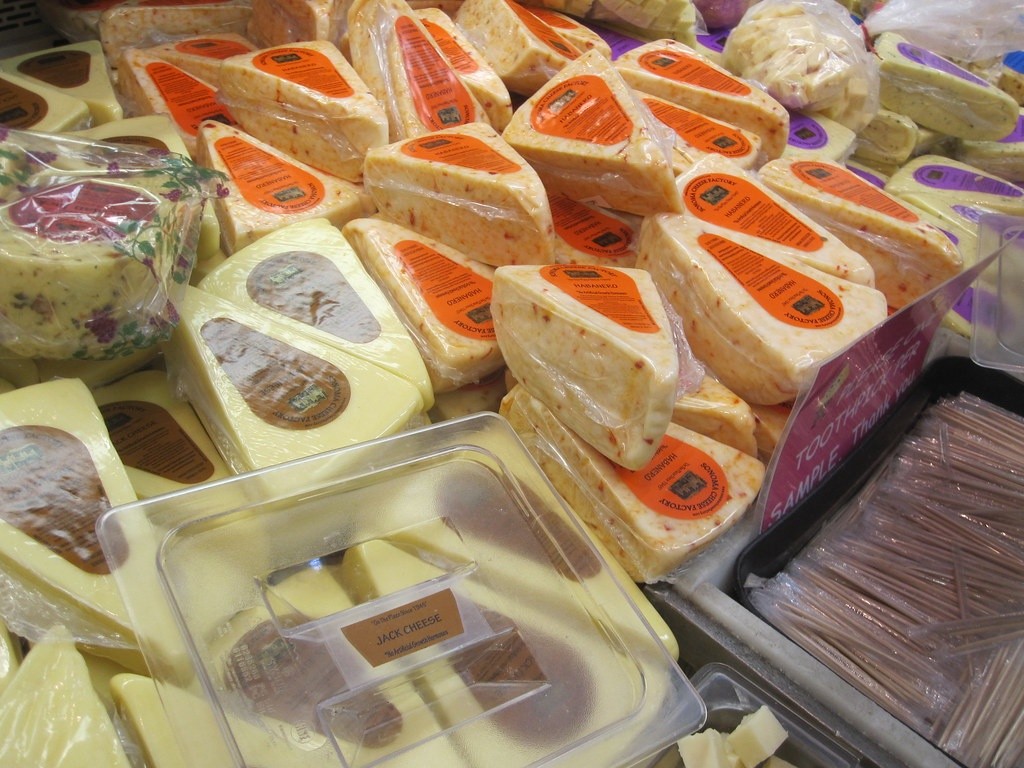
[0,0,1024,768]
